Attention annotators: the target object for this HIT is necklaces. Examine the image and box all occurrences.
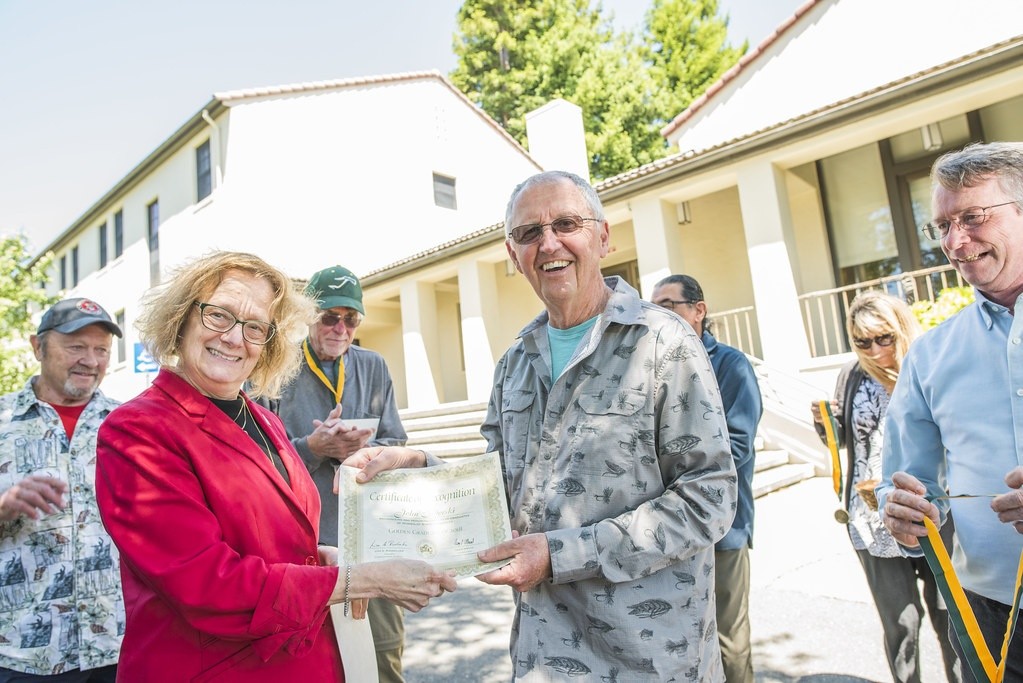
[230,395,275,467]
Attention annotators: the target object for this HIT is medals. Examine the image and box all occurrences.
[834,509,849,523]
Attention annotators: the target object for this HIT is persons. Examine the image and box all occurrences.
[96,250,457,683]
[876,140,1023,683]
[812,293,962,683]
[0,298,125,683]
[244,265,408,683]
[648,274,762,683]
[333,171,739,683]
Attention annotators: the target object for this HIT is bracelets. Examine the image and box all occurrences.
[344,565,352,616]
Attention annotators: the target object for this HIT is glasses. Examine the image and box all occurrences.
[322,311,361,328]
[921,201,1018,241]
[508,216,600,245]
[658,300,696,311]
[853,333,895,350]
[193,300,276,345]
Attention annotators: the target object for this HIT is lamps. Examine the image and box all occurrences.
[505,258,518,279]
[920,122,944,152]
[677,200,692,226]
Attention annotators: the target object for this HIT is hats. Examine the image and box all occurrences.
[37,298,123,339]
[301,265,365,315]
